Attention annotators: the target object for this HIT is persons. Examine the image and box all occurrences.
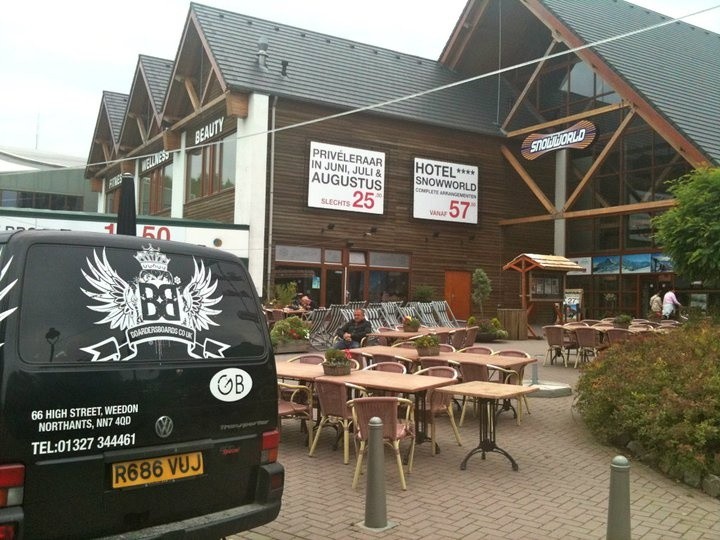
[648,288,663,318]
[661,285,683,321]
[332,308,372,349]
[300,295,319,322]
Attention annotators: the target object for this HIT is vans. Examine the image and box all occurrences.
[0,231,287,540]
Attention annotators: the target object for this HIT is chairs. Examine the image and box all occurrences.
[273,315,683,491]
